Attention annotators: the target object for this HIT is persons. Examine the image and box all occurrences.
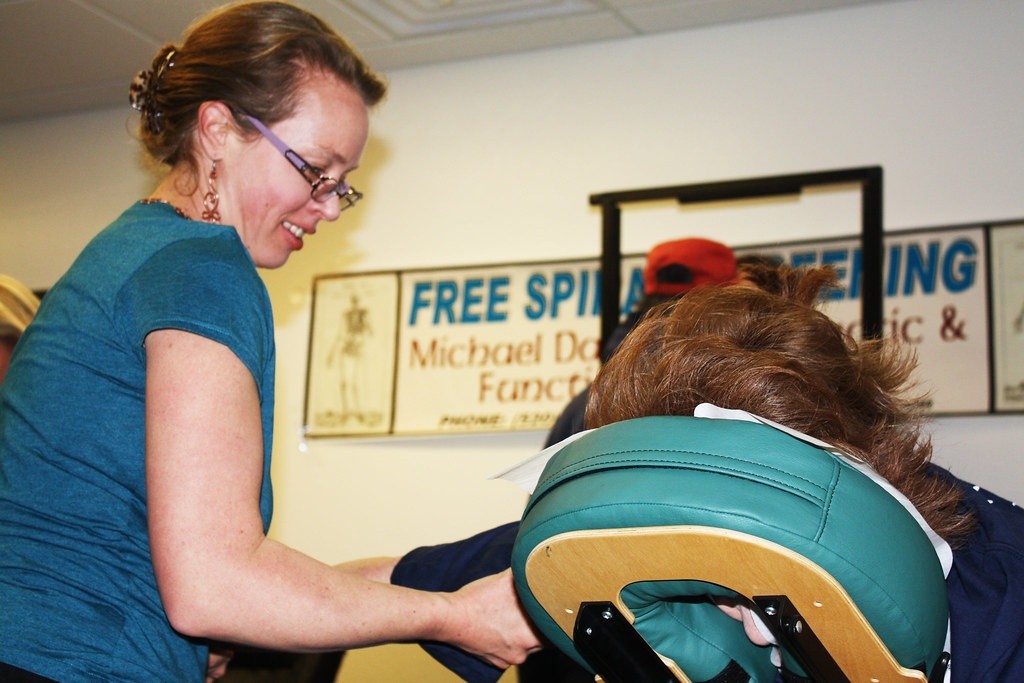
[205,237,1023,683]
[0,0,547,683]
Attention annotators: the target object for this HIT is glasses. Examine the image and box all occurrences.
[237,114,362,213]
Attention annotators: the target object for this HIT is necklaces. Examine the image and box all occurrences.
[140,198,193,220]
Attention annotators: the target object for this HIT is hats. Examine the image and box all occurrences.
[644,239,737,295]
